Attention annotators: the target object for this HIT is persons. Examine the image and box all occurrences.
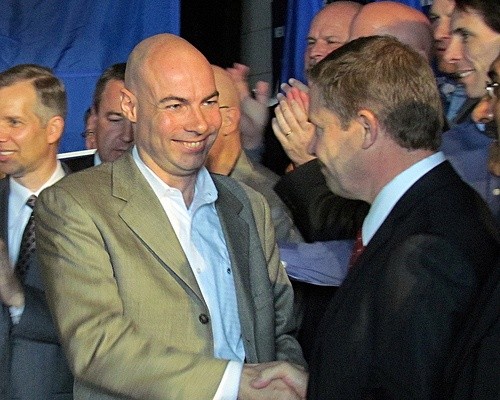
[0,64,75,400]
[249,34,500,400]
[83,0,500,360]
[35,34,308,400]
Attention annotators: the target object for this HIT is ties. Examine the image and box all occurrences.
[347,229,364,267]
[13,195,40,291]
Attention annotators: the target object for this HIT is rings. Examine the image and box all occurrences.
[285,130,291,137]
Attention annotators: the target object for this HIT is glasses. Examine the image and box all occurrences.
[80,131,96,138]
[483,78,500,100]
